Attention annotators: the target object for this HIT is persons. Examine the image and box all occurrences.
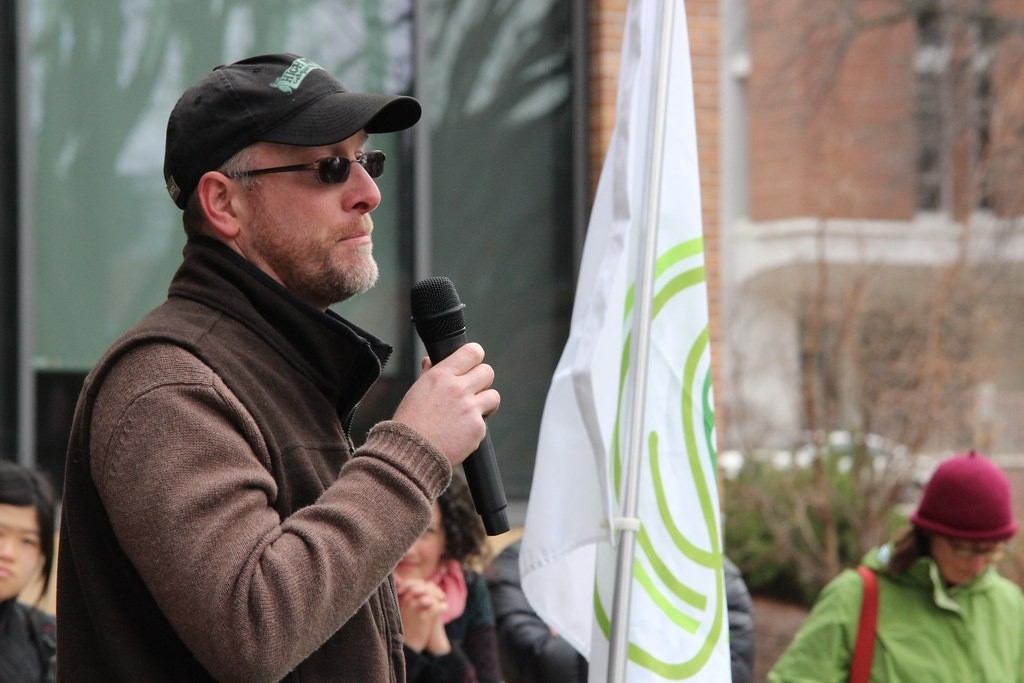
[484,534,756,683]
[384,471,496,683]
[764,448,1024,683]
[1,464,54,683]
[57,53,500,682]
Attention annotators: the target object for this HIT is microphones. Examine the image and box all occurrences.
[410,276,510,536]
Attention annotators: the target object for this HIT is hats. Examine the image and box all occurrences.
[910,449,1019,541]
[163,52,422,213]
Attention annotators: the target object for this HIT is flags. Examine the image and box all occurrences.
[518,0,733,683]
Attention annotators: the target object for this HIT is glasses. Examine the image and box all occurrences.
[937,535,1006,564]
[225,149,386,186]
